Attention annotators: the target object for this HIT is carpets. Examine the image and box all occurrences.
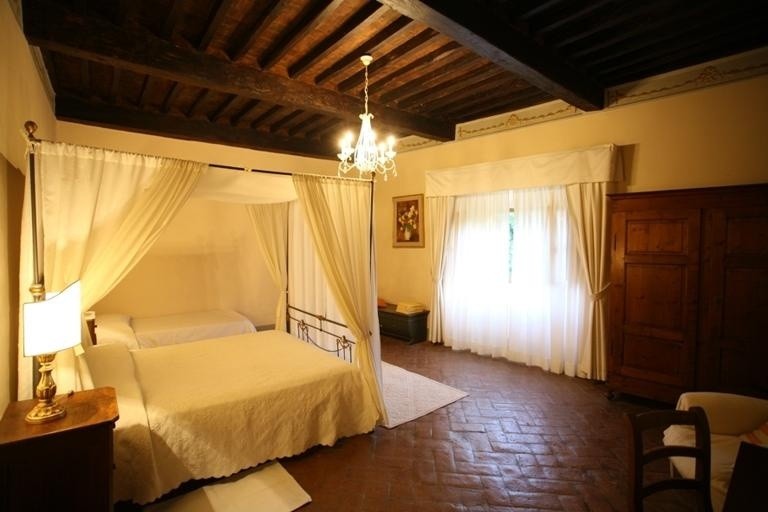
[379,360,470,430]
[145,459,313,511]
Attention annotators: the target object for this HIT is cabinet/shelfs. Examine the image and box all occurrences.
[603,184,767,401]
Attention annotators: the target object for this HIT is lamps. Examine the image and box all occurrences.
[22,300,82,425]
[337,54,398,184]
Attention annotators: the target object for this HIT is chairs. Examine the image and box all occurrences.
[622,391,768,511]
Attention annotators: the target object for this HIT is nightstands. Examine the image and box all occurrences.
[0,386,120,511]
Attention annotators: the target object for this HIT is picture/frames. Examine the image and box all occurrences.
[391,194,426,248]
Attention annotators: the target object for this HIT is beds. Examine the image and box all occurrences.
[83,306,258,350]
[50,327,381,510]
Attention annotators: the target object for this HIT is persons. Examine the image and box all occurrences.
[398,205,418,241]
